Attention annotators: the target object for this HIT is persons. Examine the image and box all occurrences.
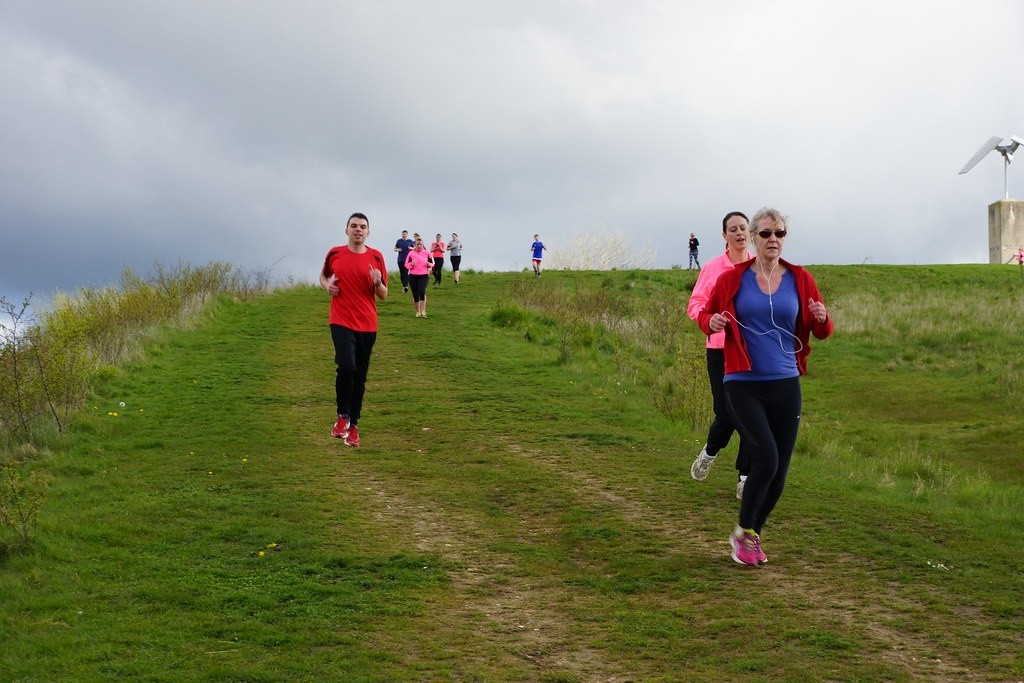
[431,233,446,286]
[1016,248,1024,265]
[404,237,435,318]
[447,233,463,284]
[531,234,546,277]
[688,233,700,269]
[698,208,835,567]
[394,230,415,292]
[687,215,756,499]
[413,233,420,239]
[319,212,388,447]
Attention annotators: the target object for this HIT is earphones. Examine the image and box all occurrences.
[752,237,755,245]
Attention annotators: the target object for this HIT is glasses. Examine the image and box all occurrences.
[755,230,787,238]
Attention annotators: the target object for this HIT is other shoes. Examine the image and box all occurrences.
[415,312,421,318]
[421,312,427,318]
[402,287,408,292]
[433,281,437,285]
[538,273,541,277]
[455,280,458,284]
[438,281,440,285]
[535,271,537,275]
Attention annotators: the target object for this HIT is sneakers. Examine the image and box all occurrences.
[690,444,718,480]
[330,414,350,438]
[344,424,360,447]
[728,531,760,565]
[755,542,768,563]
[736,476,747,499]
